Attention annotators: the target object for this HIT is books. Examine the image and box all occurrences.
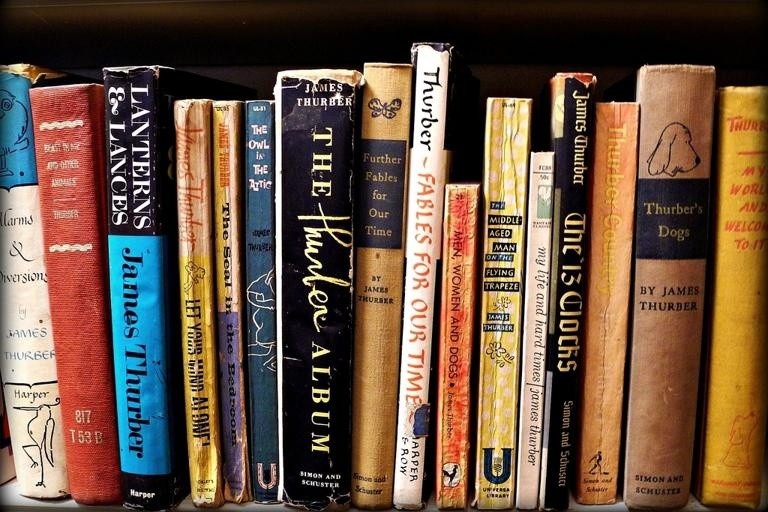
[1,41,767,512]
